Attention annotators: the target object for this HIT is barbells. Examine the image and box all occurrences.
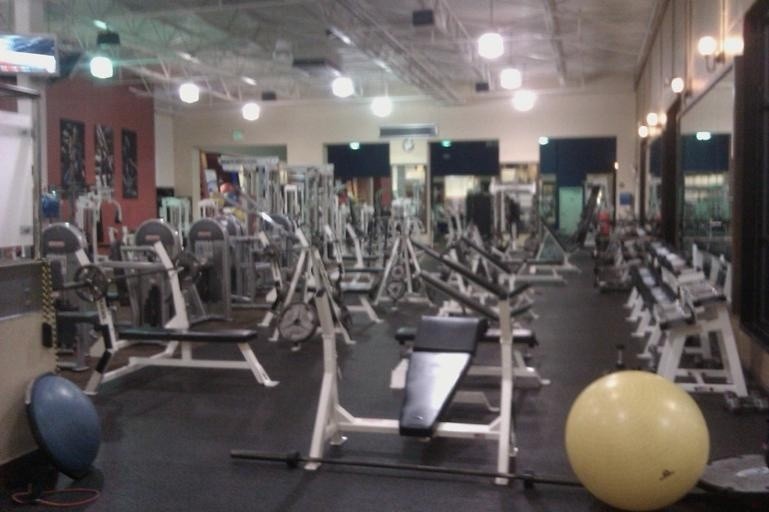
[65,253,203,302]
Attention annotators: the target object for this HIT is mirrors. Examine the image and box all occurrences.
[676,61,742,290]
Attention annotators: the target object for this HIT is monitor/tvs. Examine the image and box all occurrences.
[539,137,617,173]
[430,141,499,175]
[650,133,731,172]
[328,142,391,177]
